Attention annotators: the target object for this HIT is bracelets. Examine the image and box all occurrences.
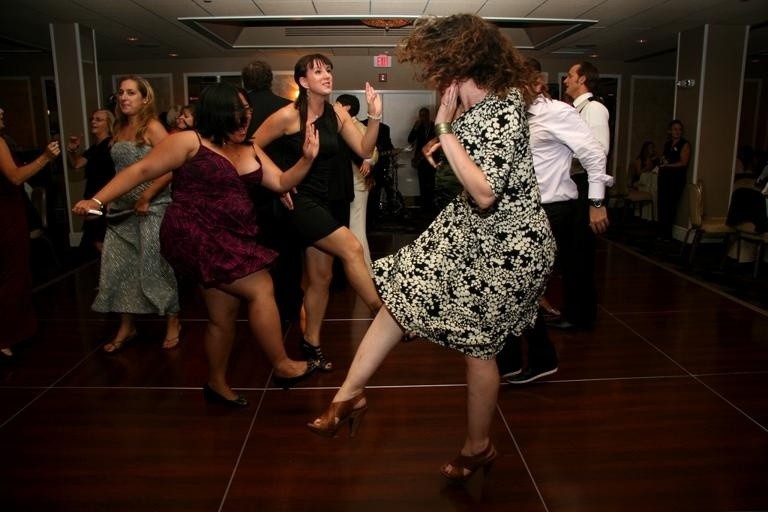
[36,159,44,169]
[434,122,454,137]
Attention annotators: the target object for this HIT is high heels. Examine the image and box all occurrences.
[440,436,500,482]
[201,382,248,409]
[304,391,370,442]
[274,356,322,386]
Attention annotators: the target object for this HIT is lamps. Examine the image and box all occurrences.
[361,18,415,31]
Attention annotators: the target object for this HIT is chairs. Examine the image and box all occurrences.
[608,163,768,278]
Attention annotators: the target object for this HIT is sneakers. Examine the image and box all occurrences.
[505,352,560,387]
[493,357,524,378]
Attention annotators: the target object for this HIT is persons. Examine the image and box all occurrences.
[0,106,62,360]
[625,140,661,191]
[62,59,397,355]
[68,79,322,409]
[305,13,559,485]
[495,55,617,386]
[247,52,420,375]
[542,60,610,331]
[655,118,693,242]
[406,105,441,210]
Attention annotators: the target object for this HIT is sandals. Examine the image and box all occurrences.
[296,341,335,373]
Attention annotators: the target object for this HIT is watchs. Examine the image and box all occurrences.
[587,198,606,208]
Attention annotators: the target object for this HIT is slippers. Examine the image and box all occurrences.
[102,326,138,354]
[162,319,183,352]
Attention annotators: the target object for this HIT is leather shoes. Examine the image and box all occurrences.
[538,317,596,331]
